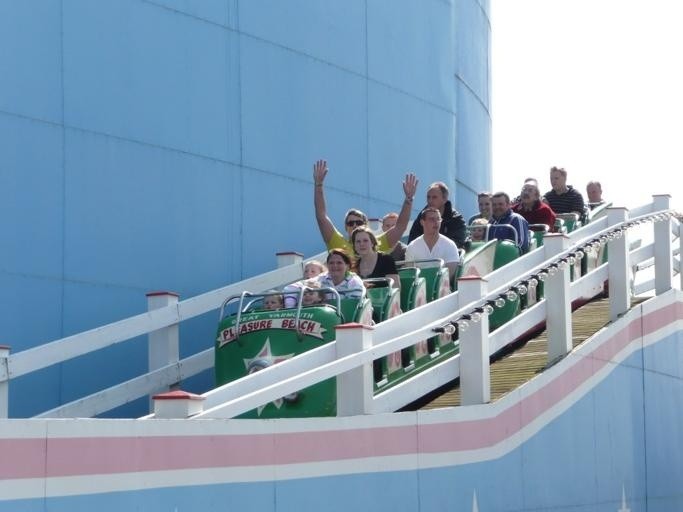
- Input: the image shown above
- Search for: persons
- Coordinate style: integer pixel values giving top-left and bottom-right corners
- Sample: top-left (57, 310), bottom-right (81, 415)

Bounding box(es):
top-left (586, 181), bottom-right (603, 209)
top-left (303, 290), bottom-right (325, 306)
top-left (381, 213), bottom-right (407, 261)
top-left (512, 182), bottom-right (556, 232)
top-left (283, 248), bottom-right (366, 309)
top-left (313, 159), bottom-right (419, 269)
top-left (511, 178), bottom-right (550, 206)
top-left (542, 165), bottom-right (583, 219)
top-left (466, 218), bottom-right (490, 245)
top-left (484, 193), bottom-right (530, 253)
top-left (350, 226), bottom-right (401, 294)
top-left (468, 193), bottom-right (494, 225)
top-left (408, 182), bottom-right (471, 249)
top-left (405, 207), bottom-right (459, 281)
top-left (304, 261), bottom-right (323, 280)
top-left (264, 290), bottom-right (282, 310)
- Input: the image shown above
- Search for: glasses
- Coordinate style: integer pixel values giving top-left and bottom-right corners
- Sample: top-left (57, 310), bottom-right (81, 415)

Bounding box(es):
top-left (346, 220), bottom-right (363, 226)
top-left (437, 218), bottom-right (443, 222)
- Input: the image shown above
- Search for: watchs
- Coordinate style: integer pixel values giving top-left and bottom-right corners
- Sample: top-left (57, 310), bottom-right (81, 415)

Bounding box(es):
top-left (314, 183), bottom-right (322, 188)
